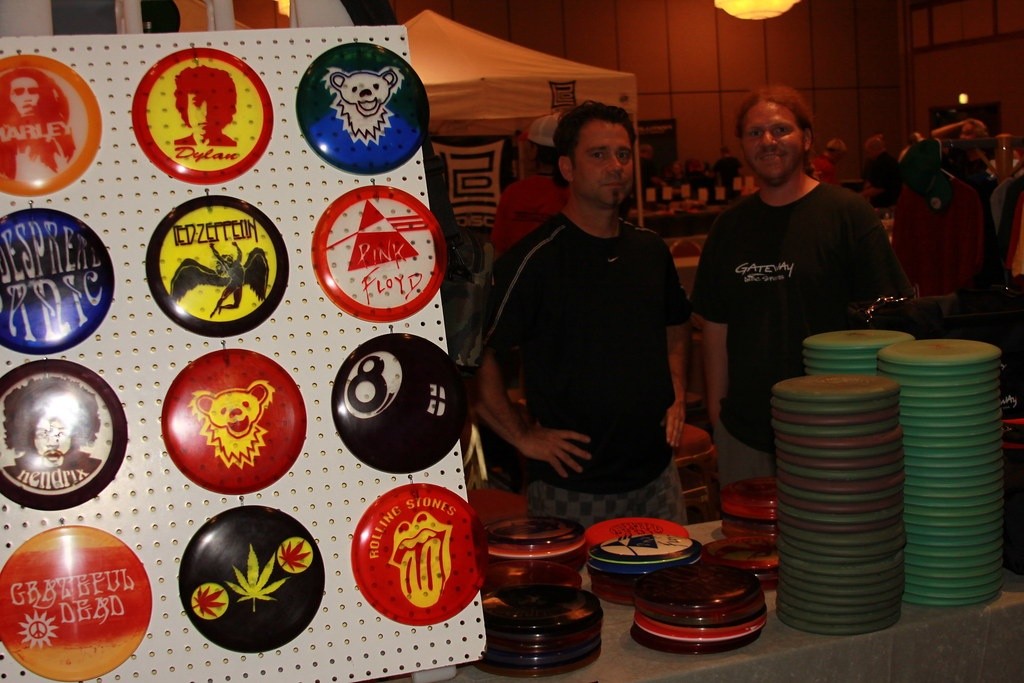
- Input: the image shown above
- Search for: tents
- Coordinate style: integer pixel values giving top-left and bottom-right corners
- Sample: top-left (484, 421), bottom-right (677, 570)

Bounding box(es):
top-left (405, 9), bottom-right (644, 231)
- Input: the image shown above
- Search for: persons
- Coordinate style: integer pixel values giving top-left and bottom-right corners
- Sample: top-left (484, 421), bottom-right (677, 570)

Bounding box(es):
top-left (814, 119), bottom-right (1024, 304)
top-left (698, 87), bottom-right (913, 480)
top-left (492, 111), bottom-right (571, 255)
top-left (638, 140), bottom-right (744, 206)
top-left (470, 98), bottom-right (694, 530)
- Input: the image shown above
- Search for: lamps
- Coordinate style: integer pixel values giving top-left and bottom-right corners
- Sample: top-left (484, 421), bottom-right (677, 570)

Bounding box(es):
top-left (714, 0), bottom-right (801, 20)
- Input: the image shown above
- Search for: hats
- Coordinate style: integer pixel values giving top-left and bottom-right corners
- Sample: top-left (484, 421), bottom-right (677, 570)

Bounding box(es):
top-left (528, 112), bottom-right (560, 146)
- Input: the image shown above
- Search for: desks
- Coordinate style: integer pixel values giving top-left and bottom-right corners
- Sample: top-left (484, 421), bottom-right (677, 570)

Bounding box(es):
top-left (660, 234), bottom-right (709, 279)
top-left (625, 202), bottom-right (727, 235)
top-left (444, 517), bottom-right (1024, 682)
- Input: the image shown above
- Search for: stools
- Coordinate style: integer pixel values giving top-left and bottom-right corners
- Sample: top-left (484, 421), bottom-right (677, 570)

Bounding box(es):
top-left (673, 423), bottom-right (723, 524)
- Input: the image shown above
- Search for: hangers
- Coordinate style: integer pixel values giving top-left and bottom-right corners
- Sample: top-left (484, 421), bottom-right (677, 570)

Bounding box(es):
top-left (933, 137), bottom-right (1024, 179)
